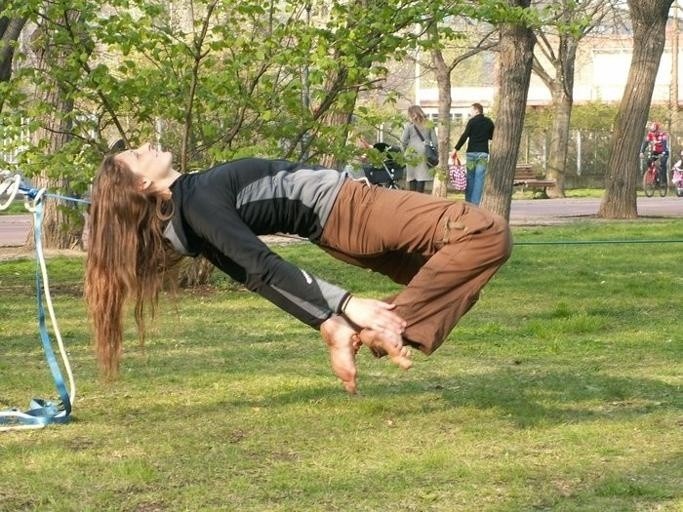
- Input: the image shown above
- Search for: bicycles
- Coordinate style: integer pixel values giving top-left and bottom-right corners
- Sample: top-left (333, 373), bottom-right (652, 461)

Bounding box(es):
top-left (639, 150), bottom-right (668, 197)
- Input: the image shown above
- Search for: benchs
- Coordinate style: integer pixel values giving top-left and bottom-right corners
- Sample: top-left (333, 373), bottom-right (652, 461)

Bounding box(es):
top-left (513, 165), bottom-right (557, 198)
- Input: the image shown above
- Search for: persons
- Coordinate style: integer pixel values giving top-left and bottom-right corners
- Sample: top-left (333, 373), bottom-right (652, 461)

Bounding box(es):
top-left (640, 122), bottom-right (670, 190)
top-left (78, 143), bottom-right (513, 398)
top-left (399, 105), bottom-right (439, 192)
top-left (669, 151), bottom-right (683, 191)
top-left (450, 103), bottom-right (494, 207)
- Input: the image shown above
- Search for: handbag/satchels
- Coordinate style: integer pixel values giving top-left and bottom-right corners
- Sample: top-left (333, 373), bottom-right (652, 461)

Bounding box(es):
top-left (446, 156), bottom-right (469, 191)
top-left (424, 143), bottom-right (441, 164)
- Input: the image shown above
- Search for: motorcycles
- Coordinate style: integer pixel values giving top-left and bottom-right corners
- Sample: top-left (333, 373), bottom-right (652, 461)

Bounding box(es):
top-left (671, 167), bottom-right (683, 197)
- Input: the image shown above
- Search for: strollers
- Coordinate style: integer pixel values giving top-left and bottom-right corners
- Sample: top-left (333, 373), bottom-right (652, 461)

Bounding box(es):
top-left (358, 136), bottom-right (409, 192)
